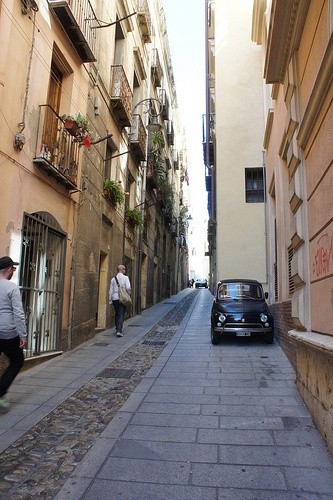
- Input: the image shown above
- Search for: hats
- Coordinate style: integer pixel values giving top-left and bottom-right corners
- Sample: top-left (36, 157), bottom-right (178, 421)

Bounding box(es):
top-left (0, 256), bottom-right (20, 269)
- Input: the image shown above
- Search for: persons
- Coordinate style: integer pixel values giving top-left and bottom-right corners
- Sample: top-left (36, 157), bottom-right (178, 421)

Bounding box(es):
top-left (0, 256), bottom-right (27, 410)
top-left (108, 265), bottom-right (131, 337)
top-left (189, 279), bottom-right (194, 288)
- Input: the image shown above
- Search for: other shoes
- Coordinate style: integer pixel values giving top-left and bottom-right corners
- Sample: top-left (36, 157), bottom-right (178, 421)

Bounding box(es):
top-left (116, 332), bottom-right (124, 337)
top-left (0, 396), bottom-right (12, 408)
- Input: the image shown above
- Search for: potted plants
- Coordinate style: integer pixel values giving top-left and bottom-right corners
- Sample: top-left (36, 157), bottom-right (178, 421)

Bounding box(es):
top-left (125, 209), bottom-right (142, 227)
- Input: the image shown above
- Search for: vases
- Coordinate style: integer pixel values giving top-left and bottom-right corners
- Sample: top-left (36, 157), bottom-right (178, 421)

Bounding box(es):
top-left (64, 120), bottom-right (78, 136)
top-left (104, 188), bottom-right (114, 202)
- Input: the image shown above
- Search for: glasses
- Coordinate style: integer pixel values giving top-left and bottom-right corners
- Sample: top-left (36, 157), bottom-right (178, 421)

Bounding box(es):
top-left (123, 268), bottom-right (127, 270)
top-left (11, 267), bottom-right (16, 271)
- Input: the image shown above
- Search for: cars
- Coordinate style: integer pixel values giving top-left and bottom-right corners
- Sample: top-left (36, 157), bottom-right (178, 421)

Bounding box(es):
top-left (210, 278), bottom-right (275, 347)
top-left (195, 278), bottom-right (207, 289)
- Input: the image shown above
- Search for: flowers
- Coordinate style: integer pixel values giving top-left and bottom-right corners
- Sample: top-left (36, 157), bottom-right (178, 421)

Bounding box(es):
top-left (56, 114), bottom-right (94, 148)
top-left (103, 177), bottom-right (125, 205)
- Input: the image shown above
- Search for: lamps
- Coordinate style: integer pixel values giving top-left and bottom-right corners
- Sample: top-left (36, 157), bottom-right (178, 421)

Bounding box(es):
top-left (133, 98), bottom-right (163, 131)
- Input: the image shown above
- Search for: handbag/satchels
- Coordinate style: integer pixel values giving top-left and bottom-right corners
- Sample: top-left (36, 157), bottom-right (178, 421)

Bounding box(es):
top-left (118, 287), bottom-right (133, 307)
